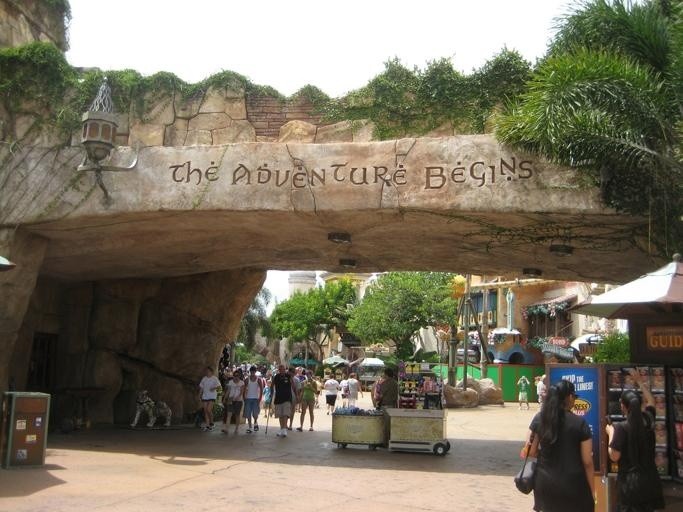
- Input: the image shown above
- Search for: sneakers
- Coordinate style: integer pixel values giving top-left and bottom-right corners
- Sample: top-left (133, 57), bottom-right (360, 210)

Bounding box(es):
top-left (221, 429), bottom-right (228, 434)
top-left (297, 427), bottom-right (303, 432)
top-left (282, 429), bottom-right (288, 438)
top-left (288, 428), bottom-right (292, 431)
top-left (309, 429), bottom-right (313, 432)
top-left (277, 431), bottom-right (281, 437)
top-left (202, 426), bottom-right (212, 433)
top-left (234, 427), bottom-right (240, 434)
top-left (209, 423), bottom-right (215, 427)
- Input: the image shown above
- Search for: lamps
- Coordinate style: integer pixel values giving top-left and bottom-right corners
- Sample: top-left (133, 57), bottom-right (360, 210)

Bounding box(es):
top-left (76, 72), bottom-right (138, 206)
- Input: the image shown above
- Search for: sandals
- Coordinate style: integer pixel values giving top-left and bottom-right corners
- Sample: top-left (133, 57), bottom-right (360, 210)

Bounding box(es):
top-left (246, 428), bottom-right (253, 433)
top-left (253, 425), bottom-right (259, 431)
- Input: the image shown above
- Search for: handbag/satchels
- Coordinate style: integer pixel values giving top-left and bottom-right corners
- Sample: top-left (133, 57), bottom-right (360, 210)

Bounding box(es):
top-left (514, 460), bottom-right (538, 495)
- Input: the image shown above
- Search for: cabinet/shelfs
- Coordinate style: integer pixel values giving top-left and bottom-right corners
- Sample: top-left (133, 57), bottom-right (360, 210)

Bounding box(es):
top-left (670, 366), bottom-right (683, 482)
top-left (605, 368), bottom-right (669, 481)
top-left (397, 362), bottom-right (438, 409)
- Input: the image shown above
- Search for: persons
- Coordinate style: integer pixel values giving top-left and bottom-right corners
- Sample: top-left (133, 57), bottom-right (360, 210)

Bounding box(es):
top-left (368, 368), bottom-right (401, 412)
top-left (196, 359), bottom-right (363, 436)
top-left (517, 376), bottom-right (530, 410)
top-left (534, 374), bottom-right (547, 403)
top-left (524, 380), bottom-right (596, 512)
top-left (605, 366), bottom-right (666, 512)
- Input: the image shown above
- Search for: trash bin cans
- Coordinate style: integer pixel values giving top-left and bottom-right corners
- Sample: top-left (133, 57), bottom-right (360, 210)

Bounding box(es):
top-left (1, 392), bottom-right (52, 469)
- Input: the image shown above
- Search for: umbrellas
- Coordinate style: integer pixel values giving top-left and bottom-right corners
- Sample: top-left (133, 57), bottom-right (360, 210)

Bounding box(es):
top-left (559, 253), bottom-right (683, 320)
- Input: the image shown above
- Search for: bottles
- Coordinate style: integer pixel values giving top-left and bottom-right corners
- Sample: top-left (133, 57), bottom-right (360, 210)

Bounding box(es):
top-left (399, 378), bottom-right (404, 393)
top-left (405, 377), bottom-right (409, 392)
top-left (412, 378), bottom-right (415, 391)
